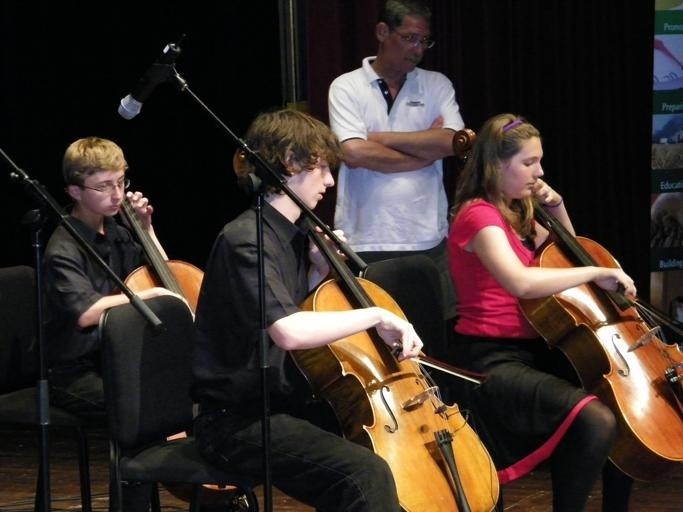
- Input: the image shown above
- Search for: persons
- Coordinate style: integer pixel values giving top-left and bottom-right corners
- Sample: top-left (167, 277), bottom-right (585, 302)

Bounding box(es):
top-left (447, 113), bottom-right (637, 512)
top-left (189, 107), bottom-right (425, 512)
top-left (328, 0), bottom-right (466, 363)
top-left (39, 136), bottom-right (195, 512)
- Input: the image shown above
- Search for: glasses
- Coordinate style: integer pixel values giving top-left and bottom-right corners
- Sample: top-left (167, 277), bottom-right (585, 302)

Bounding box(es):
top-left (393, 29), bottom-right (436, 49)
top-left (83, 178), bottom-right (130, 194)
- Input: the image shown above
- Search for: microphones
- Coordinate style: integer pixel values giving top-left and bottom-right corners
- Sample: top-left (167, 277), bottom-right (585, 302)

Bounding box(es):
top-left (117, 33), bottom-right (187, 120)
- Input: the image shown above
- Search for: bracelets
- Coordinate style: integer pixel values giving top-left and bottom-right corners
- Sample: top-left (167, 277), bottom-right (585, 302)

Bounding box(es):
top-left (543, 194), bottom-right (563, 208)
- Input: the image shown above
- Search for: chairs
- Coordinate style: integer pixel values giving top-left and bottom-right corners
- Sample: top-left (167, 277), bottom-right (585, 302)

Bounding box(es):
top-left (1, 264), bottom-right (92, 511)
top-left (100, 295), bottom-right (264, 512)
top-left (358, 254), bottom-right (453, 406)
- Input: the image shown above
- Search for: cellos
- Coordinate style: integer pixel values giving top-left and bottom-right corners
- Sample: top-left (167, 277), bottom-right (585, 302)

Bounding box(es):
top-left (233, 139), bottom-right (499, 512)
top-left (451, 128), bottom-right (683, 486)
top-left (112, 193), bottom-right (237, 504)
